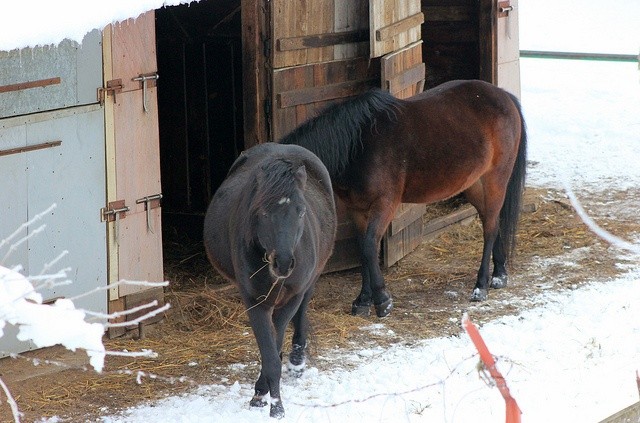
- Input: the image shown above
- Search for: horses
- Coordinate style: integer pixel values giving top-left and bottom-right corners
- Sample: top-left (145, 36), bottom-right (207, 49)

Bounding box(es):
top-left (277, 79), bottom-right (528, 318)
top-left (203, 141), bottom-right (338, 420)
